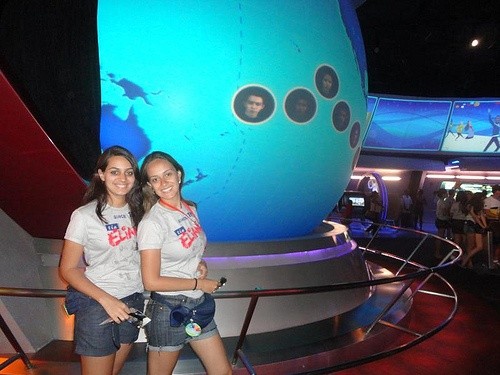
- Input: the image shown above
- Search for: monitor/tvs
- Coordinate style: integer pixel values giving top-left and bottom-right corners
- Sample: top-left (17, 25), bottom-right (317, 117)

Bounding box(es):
top-left (349, 197), bottom-right (364, 207)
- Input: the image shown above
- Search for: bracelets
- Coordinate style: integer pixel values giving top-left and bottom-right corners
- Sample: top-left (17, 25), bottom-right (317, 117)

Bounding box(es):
top-left (193, 278), bottom-right (198, 291)
top-left (136, 151), bottom-right (233, 375)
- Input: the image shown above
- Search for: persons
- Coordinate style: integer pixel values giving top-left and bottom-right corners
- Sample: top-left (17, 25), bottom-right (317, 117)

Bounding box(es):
top-left (239, 91), bottom-right (271, 123)
top-left (445, 118), bottom-right (475, 140)
top-left (317, 69), bottom-right (337, 98)
top-left (399, 190), bottom-right (425, 231)
top-left (435, 185), bottom-right (500, 269)
top-left (364, 192), bottom-right (383, 239)
top-left (289, 94), bottom-right (314, 123)
top-left (340, 194), bottom-right (354, 232)
top-left (334, 105), bottom-right (349, 131)
top-left (60, 145), bottom-right (208, 375)
top-left (482, 108), bottom-right (500, 152)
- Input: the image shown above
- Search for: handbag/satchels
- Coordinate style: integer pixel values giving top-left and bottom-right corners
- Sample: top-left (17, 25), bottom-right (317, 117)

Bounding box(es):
top-left (154, 293), bottom-right (215, 331)
top-left (62, 286), bottom-right (87, 318)
